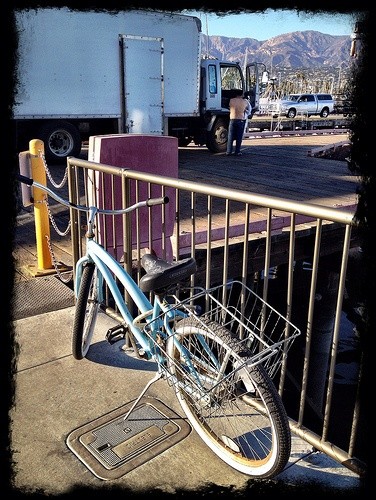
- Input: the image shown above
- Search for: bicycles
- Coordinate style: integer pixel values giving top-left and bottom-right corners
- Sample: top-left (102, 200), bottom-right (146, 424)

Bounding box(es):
top-left (18, 175), bottom-right (301, 479)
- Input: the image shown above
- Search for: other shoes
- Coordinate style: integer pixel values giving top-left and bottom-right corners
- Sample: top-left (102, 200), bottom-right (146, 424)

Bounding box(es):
top-left (225, 152), bottom-right (232, 156)
top-left (235, 152), bottom-right (243, 155)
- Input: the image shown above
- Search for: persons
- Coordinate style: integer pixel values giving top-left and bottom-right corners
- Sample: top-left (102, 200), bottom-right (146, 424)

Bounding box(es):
top-left (225, 89), bottom-right (248, 156)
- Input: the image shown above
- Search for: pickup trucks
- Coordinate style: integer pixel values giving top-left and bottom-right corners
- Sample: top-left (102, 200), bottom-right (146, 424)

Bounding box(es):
top-left (269, 93), bottom-right (334, 118)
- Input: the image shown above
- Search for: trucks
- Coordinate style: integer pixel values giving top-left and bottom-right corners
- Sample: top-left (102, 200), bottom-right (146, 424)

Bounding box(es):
top-left (13, 7), bottom-right (269, 165)
